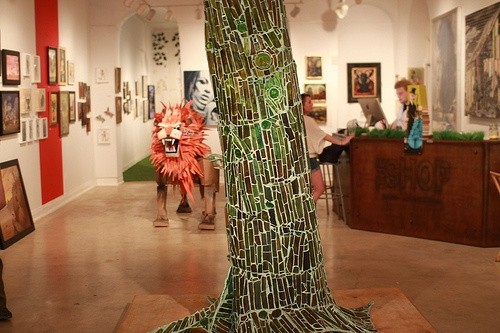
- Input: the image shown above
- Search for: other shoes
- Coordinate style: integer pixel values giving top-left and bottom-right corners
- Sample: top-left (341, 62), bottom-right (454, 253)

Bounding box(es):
top-left (0, 308), bottom-right (12, 320)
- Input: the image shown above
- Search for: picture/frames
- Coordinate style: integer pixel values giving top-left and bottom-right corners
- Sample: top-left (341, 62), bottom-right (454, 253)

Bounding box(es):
top-left (0, 158), bottom-right (35, 250)
top-left (305, 84), bottom-right (327, 102)
top-left (305, 56), bottom-right (323, 79)
top-left (184, 70), bottom-right (219, 126)
top-left (305, 106), bottom-right (326, 124)
top-left (347, 63), bottom-right (381, 104)
top-left (0, 46), bottom-right (155, 143)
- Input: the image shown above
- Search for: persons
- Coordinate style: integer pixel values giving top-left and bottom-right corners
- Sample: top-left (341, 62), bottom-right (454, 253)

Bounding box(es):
top-left (301, 94), bottom-right (354, 205)
top-left (0, 258), bottom-right (13, 319)
top-left (185, 71), bottom-right (219, 125)
top-left (394, 79), bottom-right (413, 131)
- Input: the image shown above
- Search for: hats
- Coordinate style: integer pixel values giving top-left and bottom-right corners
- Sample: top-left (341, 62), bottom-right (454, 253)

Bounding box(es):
top-left (409, 87), bottom-right (416, 95)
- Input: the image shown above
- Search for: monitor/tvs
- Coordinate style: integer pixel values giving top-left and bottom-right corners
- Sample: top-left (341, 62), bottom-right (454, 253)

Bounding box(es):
top-left (358, 98), bottom-right (389, 131)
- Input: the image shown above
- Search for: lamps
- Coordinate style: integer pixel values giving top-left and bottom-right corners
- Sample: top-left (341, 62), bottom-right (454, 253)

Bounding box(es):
top-left (135, 0), bottom-right (350, 21)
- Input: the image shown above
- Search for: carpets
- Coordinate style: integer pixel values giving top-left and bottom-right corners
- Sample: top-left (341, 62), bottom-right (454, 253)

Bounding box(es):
top-left (123, 153), bottom-right (202, 182)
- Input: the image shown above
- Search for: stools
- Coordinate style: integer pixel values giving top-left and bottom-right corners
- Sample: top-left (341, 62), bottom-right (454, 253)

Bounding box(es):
top-left (319, 160), bottom-right (346, 224)
